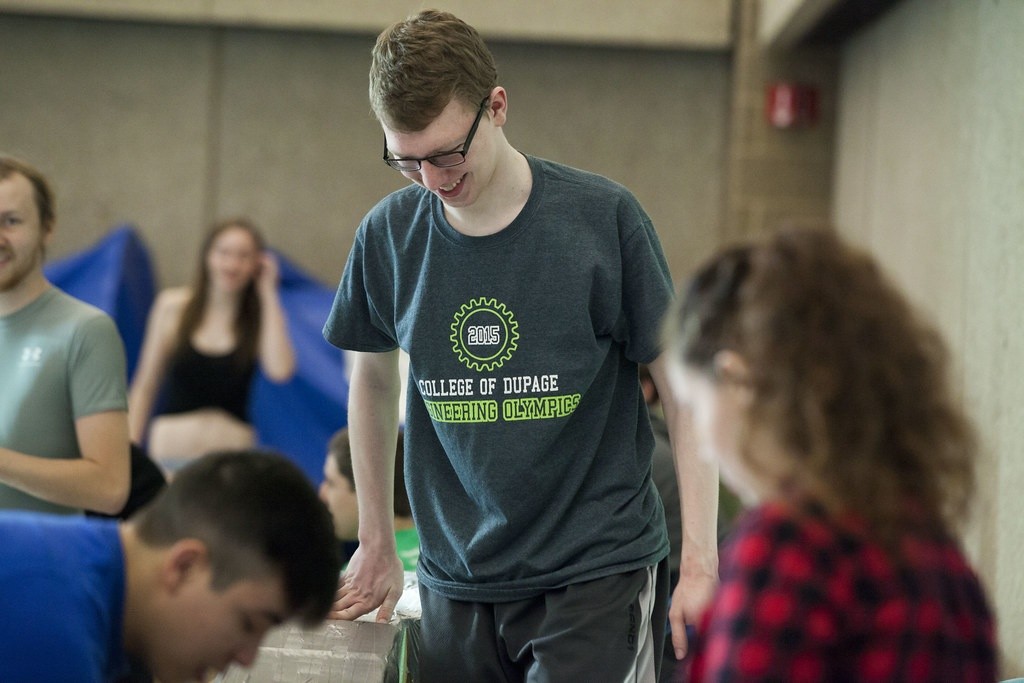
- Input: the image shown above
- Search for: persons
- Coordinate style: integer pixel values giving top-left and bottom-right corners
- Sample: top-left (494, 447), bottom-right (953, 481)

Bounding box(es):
top-left (319, 8), bottom-right (720, 683)
top-left (0, 153), bottom-right (419, 683)
top-left (665, 222), bottom-right (1003, 683)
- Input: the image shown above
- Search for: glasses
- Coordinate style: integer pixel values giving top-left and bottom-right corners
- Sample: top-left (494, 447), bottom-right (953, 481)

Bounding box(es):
top-left (382, 96), bottom-right (489, 172)
